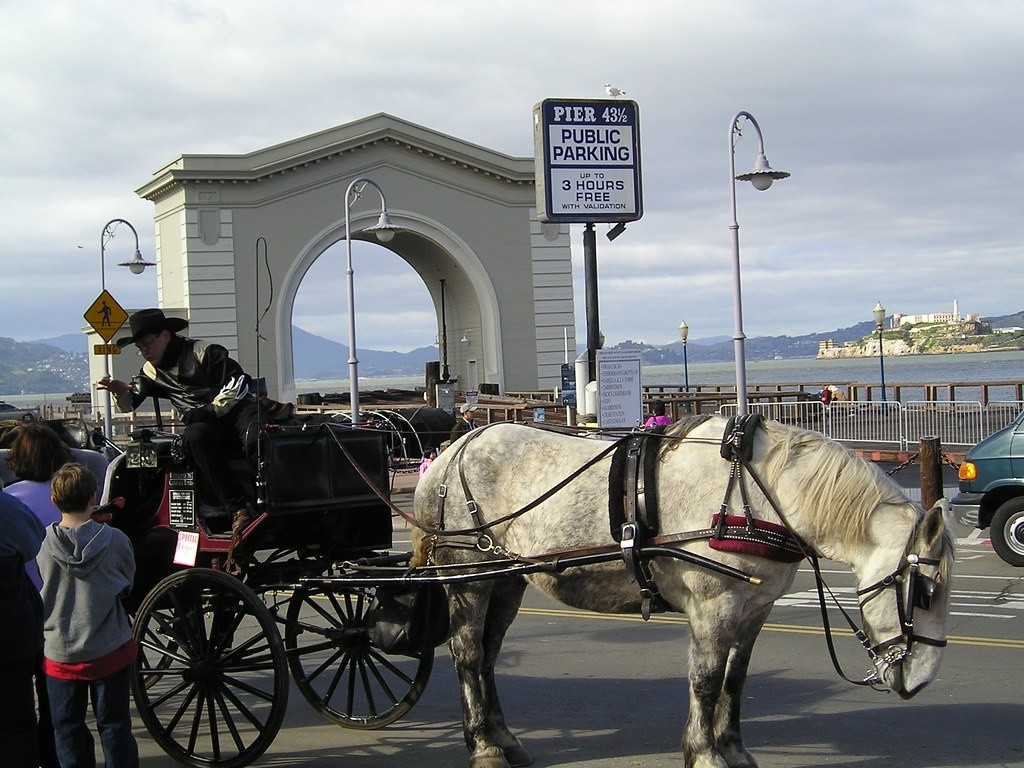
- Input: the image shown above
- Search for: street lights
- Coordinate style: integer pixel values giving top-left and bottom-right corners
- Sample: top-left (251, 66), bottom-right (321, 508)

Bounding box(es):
top-left (678, 320), bottom-right (692, 412)
top-left (101, 218), bottom-right (156, 447)
top-left (872, 301), bottom-right (888, 415)
top-left (344, 178), bottom-right (409, 429)
top-left (729, 111), bottom-right (791, 418)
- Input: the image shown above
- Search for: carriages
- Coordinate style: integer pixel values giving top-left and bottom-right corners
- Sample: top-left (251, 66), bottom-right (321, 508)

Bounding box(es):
top-left (0, 376), bottom-right (954, 768)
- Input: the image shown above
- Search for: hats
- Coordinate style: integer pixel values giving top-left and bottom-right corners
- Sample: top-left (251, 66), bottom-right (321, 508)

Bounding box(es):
top-left (116, 308), bottom-right (189, 349)
top-left (460, 402), bottom-right (477, 413)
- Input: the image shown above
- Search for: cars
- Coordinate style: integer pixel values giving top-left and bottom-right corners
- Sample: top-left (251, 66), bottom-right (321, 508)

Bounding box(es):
top-left (0, 401), bottom-right (40, 421)
top-left (952, 410), bottom-right (1024, 567)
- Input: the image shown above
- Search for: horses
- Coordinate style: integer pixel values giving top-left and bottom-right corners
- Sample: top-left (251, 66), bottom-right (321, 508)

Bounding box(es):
top-left (408, 414), bottom-right (962, 768)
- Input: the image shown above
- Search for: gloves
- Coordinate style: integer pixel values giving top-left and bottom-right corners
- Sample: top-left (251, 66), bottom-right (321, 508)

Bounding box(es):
top-left (182, 406), bottom-right (217, 425)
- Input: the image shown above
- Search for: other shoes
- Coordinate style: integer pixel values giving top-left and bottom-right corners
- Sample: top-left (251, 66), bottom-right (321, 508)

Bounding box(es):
top-left (232, 509), bottom-right (250, 530)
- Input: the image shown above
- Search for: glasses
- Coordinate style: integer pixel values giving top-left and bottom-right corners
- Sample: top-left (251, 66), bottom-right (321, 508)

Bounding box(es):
top-left (136, 333), bottom-right (159, 356)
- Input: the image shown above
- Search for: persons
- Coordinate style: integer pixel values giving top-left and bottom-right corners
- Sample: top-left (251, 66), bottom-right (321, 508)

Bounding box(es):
top-left (818, 384), bottom-right (847, 405)
top-left (419, 446), bottom-right (437, 479)
top-left (95, 307), bottom-right (296, 533)
top-left (0, 421), bottom-right (139, 768)
top-left (646, 400), bottom-right (671, 427)
top-left (450, 403), bottom-right (479, 447)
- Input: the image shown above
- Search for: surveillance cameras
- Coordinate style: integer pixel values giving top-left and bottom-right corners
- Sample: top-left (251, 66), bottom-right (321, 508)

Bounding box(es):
top-left (607, 224), bottom-right (627, 241)
